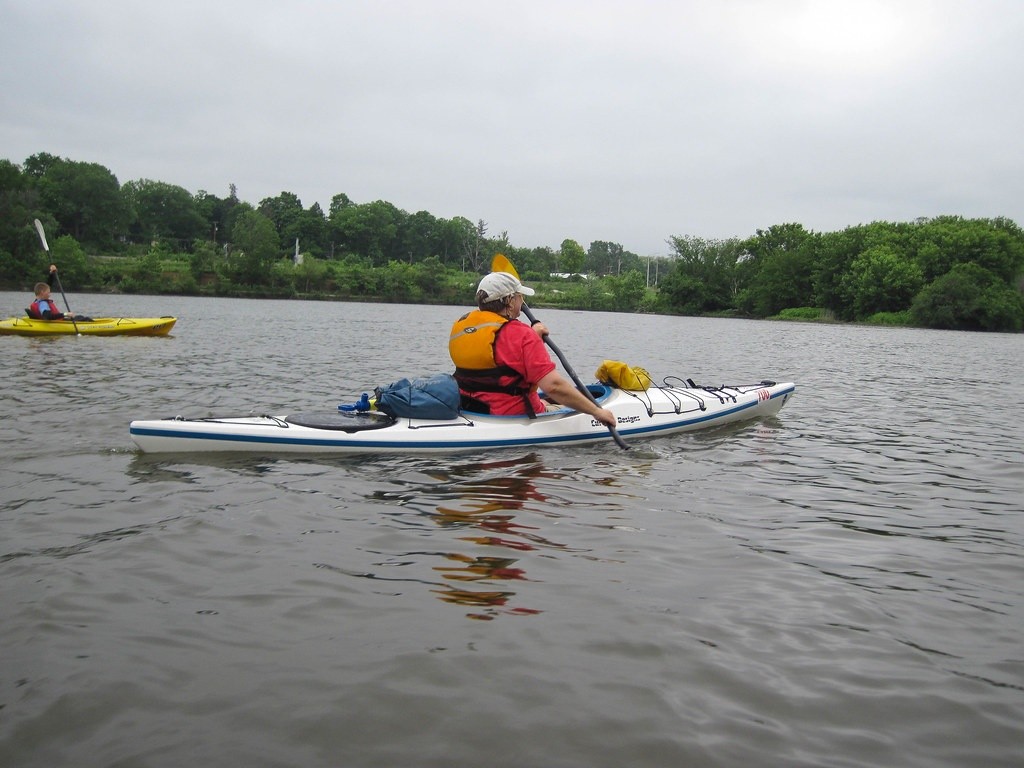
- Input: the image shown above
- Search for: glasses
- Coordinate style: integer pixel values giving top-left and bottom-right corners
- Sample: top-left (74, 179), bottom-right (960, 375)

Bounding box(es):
top-left (511, 294), bottom-right (525, 300)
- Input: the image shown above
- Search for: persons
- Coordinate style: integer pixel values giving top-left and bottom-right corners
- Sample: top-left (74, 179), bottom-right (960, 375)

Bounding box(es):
top-left (449, 272), bottom-right (616, 427)
top-left (30, 265), bottom-right (74, 320)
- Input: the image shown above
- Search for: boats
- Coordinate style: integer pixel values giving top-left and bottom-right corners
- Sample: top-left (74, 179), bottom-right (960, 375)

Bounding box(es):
top-left (0, 314), bottom-right (179, 337)
top-left (129, 358), bottom-right (799, 455)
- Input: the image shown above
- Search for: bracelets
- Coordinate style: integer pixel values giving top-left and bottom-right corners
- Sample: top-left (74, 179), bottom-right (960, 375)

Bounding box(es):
top-left (63, 312), bottom-right (68, 317)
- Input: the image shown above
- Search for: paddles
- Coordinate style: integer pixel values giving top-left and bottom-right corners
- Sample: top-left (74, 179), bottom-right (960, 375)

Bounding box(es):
top-left (34, 218), bottom-right (81, 337)
top-left (492, 253), bottom-right (634, 452)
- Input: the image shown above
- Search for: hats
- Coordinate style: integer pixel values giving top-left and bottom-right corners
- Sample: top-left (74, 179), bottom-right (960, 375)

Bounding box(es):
top-left (476, 272), bottom-right (535, 303)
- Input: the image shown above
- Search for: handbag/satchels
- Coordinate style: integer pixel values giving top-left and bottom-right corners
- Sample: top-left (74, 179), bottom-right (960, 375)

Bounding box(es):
top-left (374, 373), bottom-right (460, 419)
